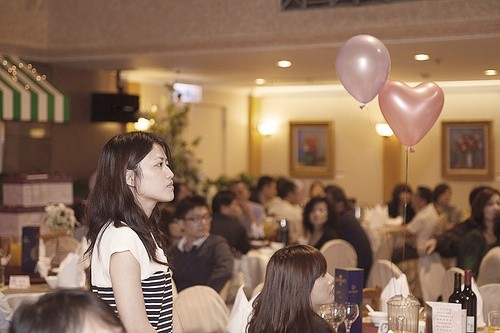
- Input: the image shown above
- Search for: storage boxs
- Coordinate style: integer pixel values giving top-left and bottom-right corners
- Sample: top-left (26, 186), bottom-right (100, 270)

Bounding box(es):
top-left (334, 268), bottom-right (365, 333)
top-left (21, 225), bottom-right (40, 277)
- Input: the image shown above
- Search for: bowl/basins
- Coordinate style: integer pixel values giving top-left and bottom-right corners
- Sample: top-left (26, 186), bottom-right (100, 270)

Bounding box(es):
top-left (367, 311), bottom-right (388, 326)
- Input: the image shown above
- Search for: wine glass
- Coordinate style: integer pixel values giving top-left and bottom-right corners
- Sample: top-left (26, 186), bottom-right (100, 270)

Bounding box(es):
top-left (316, 301), bottom-right (359, 333)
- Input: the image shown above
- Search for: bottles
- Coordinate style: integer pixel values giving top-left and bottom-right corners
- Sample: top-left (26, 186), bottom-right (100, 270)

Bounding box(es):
top-left (462, 270), bottom-right (477, 333)
top-left (448, 272), bottom-right (468, 333)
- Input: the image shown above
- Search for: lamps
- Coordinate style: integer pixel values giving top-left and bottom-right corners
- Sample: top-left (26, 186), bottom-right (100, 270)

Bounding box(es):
top-left (375, 122), bottom-right (394, 137)
top-left (134, 117), bottom-right (155, 131)
top-left (256, 123), bottom-right (277, 137)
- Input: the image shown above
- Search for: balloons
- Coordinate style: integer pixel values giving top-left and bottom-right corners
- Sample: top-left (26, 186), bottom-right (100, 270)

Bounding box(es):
top-left (337, 34), bottom-right (390, 104)
top-left (380, 79), bottom-right (444, 146)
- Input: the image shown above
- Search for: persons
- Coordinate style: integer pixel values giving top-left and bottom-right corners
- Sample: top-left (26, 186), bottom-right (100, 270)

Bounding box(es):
top-left (9, 289), bottom-right (126, 333)
top-left (84, 131), bottom-right (173, 333)
top-left (158, 183), bottom-right (234, 294)
top-left (210, 189), bottom-right (251, 258)
top-left (229, 180), bottom-right (263, 239)
top-left (267, 176), bottom-right (373, 289)
top-left (388, 183), bottom-right (500, 281)
top-left (250, 175), bottom-right (276, 205)
top-left (228, 245), bottom-right (336, 333)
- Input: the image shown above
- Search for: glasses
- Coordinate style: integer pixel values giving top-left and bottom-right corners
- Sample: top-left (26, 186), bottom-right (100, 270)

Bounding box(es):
top-left (187, 215), bottom-right (214, 224)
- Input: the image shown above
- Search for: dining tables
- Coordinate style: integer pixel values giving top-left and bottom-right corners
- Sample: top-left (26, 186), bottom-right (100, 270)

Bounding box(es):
top-left (1, 282), bottom-right (56, 330)
top-left (361, 310), bottom-right (489, 333)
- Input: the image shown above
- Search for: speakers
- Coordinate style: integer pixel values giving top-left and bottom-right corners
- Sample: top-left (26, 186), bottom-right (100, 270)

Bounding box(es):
top-left (91, 93), bottom-right (139, 122)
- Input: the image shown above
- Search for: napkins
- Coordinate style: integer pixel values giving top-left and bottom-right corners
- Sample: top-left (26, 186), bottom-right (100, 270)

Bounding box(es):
top-left (461, 276), bottom-right (487, 329)
top-left (379, 273), bottom-right (410, 312)
top-left (225, 282), bottom-right (261, 333)
top-left (32, 238), bottom-right (55, 279)
top-left (56, 252), bottom-right (87, 289)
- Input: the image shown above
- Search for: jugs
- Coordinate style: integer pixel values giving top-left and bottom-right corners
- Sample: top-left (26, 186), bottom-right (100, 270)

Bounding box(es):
top-left (386, 294), bottom-right (419, 333)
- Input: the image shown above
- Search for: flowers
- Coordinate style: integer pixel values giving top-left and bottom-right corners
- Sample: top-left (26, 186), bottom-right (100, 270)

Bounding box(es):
top-left (43, 201), bottom-right (81, 239)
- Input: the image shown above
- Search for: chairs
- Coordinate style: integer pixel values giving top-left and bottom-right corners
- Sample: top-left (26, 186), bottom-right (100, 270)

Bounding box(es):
top-left (43, 218), bottom-right (500, 333)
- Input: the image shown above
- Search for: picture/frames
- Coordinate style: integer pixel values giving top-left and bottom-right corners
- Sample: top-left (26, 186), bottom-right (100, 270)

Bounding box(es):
top-left (440, 119), bottom-right (496, 183)
top-left (288, 121), bottom-right (336, 180)
